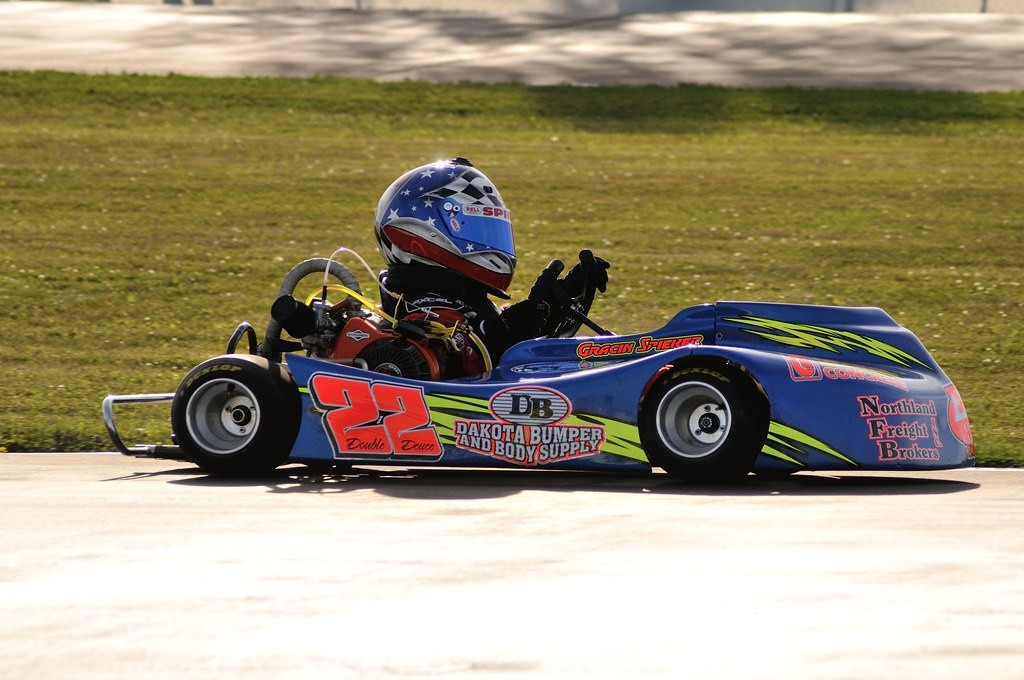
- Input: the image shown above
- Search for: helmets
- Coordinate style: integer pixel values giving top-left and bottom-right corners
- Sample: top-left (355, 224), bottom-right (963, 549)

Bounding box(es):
top-left (373, 156), bottom-right (516, 300)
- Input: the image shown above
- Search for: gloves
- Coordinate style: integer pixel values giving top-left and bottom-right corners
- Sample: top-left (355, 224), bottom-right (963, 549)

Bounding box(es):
top-left (557, 250), bottom-right (610, 336)
top-left (501, 258), bottom-right (577, 340)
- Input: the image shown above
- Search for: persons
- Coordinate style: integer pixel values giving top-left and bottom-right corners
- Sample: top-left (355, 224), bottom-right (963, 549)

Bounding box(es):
top-left (373, 154), bottom-right (610, 369)
